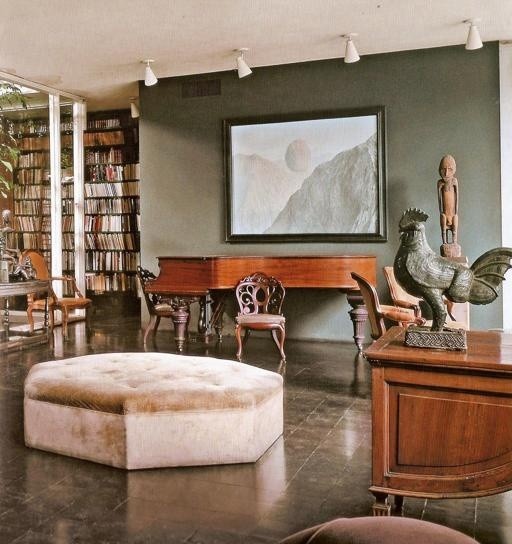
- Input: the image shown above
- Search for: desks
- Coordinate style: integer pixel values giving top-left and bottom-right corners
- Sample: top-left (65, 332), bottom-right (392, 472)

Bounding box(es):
top-left (0, 280), bottom-right (49, 354)
top-left (363, 326), bottom-right (512, 516)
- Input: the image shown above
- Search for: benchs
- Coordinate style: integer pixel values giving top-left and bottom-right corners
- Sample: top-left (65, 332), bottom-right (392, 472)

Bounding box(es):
top-left (23, 352), bottom-right (283, 470)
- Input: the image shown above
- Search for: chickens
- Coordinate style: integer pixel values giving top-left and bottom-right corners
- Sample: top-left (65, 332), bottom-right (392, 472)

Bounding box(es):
top-left (392, 206), bottom-right (512, 333)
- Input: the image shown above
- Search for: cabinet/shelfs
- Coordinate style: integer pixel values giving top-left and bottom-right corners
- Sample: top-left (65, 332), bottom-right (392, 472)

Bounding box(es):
top-left (12, 108), bottom-right (140, 314)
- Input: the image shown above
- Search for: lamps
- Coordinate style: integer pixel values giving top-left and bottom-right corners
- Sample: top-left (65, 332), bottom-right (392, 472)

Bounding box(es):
top-left (128, 97), bottom-right (140, 118)
top-left (344, 33), bottom-right (360, 64)
top-left (464, 17), bottom-right (483, 51)
top-left (141, 60), bottom-right (158, 86)
top-left (233, 48), bottom-right (253, 79)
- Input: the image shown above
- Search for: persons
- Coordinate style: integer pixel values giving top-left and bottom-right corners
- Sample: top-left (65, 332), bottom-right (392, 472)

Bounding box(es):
top-left (436, 155), bottom-right (460, 245)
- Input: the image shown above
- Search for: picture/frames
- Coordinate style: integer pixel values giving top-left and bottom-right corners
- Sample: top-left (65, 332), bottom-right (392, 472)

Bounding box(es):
top-left (223, 106), bottom-right (388, 243)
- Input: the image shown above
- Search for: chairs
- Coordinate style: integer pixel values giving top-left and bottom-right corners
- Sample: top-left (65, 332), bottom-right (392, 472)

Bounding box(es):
top-left (351, 272), bottom-right (426, 344)
top-left (19, 249), bottom-right (92, 342)
top-left (235, 272), bottom-right (286, 363)
top-left (382, 266), bottom-right (465, 329)
top-left (136, 266), bottom-right (191, 345)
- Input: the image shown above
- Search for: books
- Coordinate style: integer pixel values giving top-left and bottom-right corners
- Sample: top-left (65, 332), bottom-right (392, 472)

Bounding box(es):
top-left (10, 117), bottom-right (139, 294)
